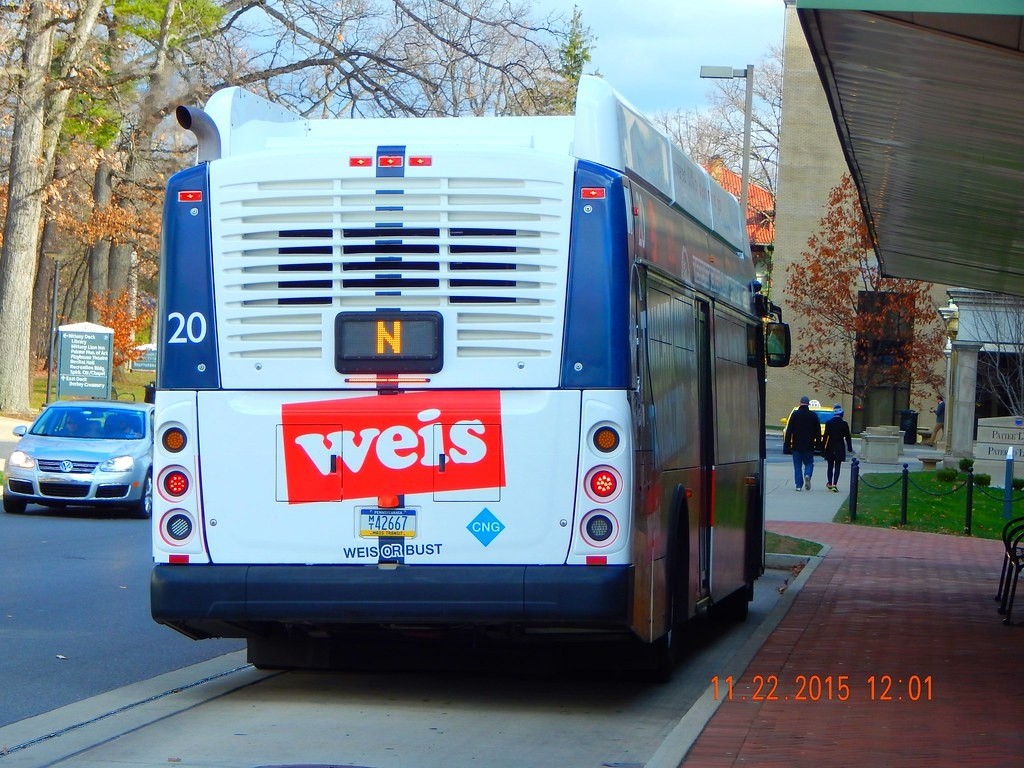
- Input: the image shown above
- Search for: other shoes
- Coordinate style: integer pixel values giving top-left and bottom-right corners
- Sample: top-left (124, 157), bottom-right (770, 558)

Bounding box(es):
top-left (796, 487), bottom-right (802, 491)
top-left (804, 475), bottom-right (811, 490)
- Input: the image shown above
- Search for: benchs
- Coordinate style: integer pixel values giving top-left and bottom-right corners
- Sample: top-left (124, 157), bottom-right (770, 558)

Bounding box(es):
top-left (994, 516), bottom-right (1024, 625)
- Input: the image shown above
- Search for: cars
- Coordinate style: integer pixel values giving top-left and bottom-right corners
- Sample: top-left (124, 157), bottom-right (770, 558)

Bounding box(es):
top-left (781, 399), bottom-right (834, 455)
top-left (2, 399), bottom-right (152, 520)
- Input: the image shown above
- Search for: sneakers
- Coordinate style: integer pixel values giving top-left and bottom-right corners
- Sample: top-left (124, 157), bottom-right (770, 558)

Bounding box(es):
top-left (832, 486), bottom-right (839, 492)
top-left (826, 482), bottom-right (832, 489)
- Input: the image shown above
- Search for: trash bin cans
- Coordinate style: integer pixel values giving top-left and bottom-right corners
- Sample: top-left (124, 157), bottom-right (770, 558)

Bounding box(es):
top-left (900, 411), bottom-right (919, 445)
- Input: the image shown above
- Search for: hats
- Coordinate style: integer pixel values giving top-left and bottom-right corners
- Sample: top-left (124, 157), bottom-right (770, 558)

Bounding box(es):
top-left (833, 403), bottom-right (844, 418)
top-left (800, 396), bottom-right (809, 405)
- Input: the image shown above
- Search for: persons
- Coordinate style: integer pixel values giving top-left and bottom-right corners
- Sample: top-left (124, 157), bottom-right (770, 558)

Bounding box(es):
top-left (821, 403), bottom-right (853, 492)
top-left (114, 413), bottom-right (142, 439)
top-left (925, 395), bottom-right (945, 446)
top-left (784, 395), bottom-right (821, 491)
top-left (58, 410), bottom-right (90, 436)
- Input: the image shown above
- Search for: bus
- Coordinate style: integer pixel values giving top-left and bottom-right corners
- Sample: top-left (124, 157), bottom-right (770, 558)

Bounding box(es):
top-left (151, 76), bottom-right (796, 687)
